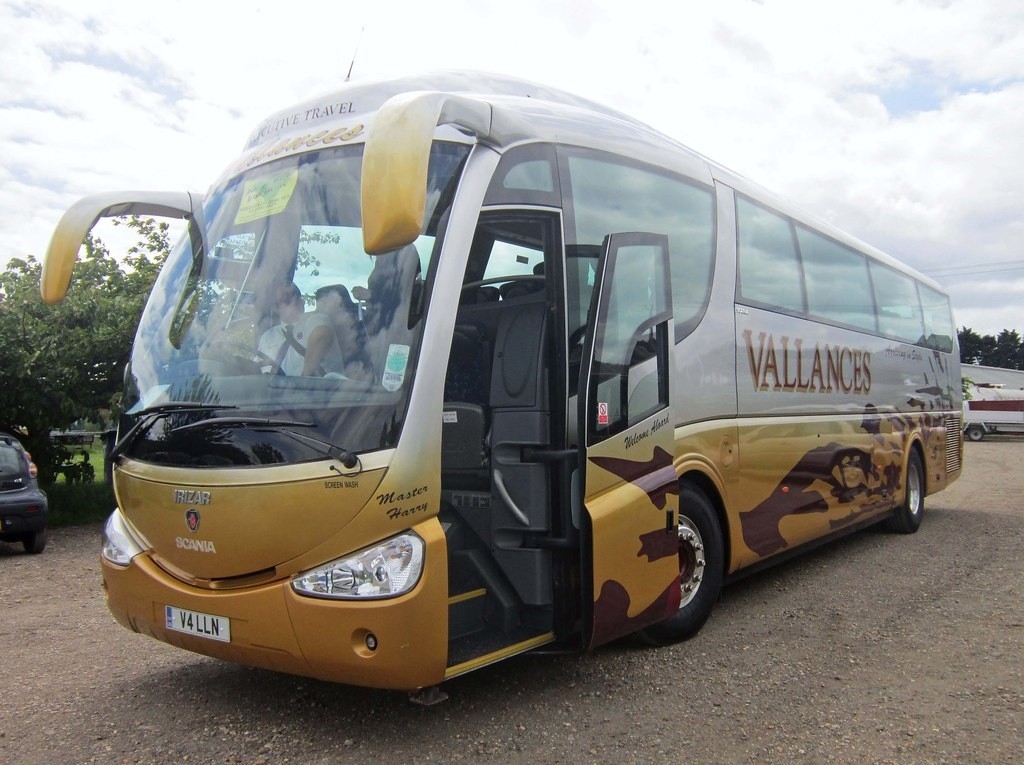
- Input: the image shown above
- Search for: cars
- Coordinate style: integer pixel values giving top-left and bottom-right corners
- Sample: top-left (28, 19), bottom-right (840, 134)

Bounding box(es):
top-left (0, 433), bottom-right (51, 555)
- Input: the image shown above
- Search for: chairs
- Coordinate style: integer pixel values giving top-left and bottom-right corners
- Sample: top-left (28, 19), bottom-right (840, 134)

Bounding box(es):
top-left (315, 262), bottom-right (589, 470)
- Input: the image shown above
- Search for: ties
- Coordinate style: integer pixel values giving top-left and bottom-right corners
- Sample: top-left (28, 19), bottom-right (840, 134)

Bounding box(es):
top-left (270, 323), bottom-right (294, 375)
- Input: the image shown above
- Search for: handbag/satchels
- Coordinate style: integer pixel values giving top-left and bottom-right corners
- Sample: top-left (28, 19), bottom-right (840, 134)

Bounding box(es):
top-left (379, 246), bottom-right (422, 330)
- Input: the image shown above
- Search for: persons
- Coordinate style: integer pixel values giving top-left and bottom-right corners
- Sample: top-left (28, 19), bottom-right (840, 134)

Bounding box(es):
top-left (251, 281), bottom-right (344, 380)
top-left (350, 241), bottom-right (422, 344)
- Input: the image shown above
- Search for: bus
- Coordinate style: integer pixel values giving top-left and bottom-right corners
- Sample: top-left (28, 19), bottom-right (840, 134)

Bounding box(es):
top-left (39, 68), bottom-right (964, 693)
top-left (959, 362), bottom-right (1024, 442)
